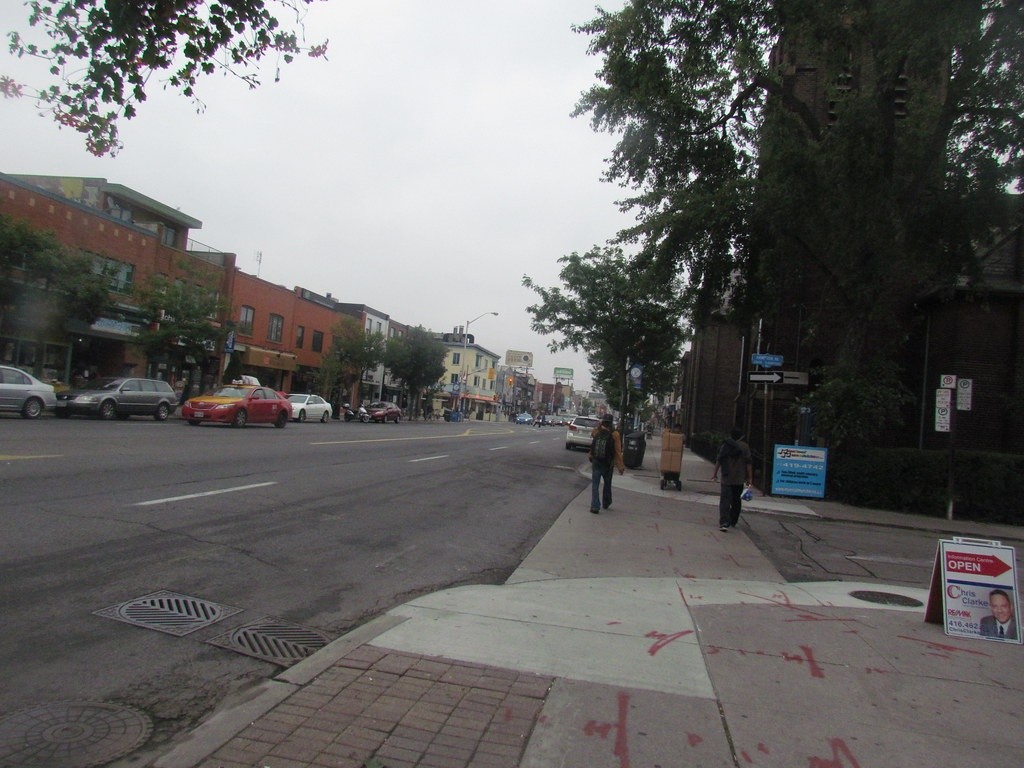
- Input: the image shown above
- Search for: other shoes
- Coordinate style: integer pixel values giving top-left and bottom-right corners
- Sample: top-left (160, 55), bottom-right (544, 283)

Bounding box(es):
top-left (603, 503), bottom-right (609, 508)
top-left (590, 509), bottom-right (599, 514)
top-left (719, 522), bottom-right (728, 531)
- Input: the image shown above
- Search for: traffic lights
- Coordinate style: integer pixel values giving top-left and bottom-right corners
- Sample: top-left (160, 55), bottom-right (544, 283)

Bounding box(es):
top-left (508, 376), bottom-right (513, 389)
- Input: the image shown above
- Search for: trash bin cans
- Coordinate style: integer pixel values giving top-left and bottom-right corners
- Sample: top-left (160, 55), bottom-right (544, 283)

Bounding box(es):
top-left (625, 431), bottom-right (647, 468)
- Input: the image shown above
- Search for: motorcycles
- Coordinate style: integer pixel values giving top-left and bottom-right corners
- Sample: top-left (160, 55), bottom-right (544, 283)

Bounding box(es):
top-left (342, 400), bottom-right (369, 423)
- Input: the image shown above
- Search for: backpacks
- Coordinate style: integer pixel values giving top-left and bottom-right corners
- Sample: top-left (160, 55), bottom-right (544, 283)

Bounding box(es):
top-left (590, 427), bottom-right (615, 464)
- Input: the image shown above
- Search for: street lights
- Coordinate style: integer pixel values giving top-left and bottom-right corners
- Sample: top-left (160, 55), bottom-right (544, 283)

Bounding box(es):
top-left (459, 312), bottom-right (499, 384)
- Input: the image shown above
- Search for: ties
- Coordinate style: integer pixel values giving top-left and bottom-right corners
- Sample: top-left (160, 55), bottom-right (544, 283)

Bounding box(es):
top-left (999, 625), bottom-right (1004, 638)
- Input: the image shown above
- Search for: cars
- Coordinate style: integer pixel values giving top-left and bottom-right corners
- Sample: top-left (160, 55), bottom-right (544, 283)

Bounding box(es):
top-left (564, 415), bottom-right (600, 451)
top-left (283, 394), bottom-right (333, 424)
top-left (536, 412), bottom-right (579, 427)
top-left (56, 376), bottom-right (180, 420)
top-left (363, 401), bottom-right (402, 423)
top-left (515, 412), bottom-right (534, 426)
top-left (0, 365), bottom-right (57, 419)
top-left (180, 379), bottom-right (293, 429)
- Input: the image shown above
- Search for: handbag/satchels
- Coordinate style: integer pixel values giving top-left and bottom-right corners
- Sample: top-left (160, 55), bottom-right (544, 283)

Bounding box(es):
top-left (740, 484), bottom-right (753, 501)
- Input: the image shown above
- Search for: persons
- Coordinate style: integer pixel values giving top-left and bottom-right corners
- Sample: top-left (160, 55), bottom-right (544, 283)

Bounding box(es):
top-left (647, 423), bottom-right (653, 432)
top-left (710, 427), bottom-right (752, 532)
top-left (980, 589), bottom-right (1018, 641)
top-left (671, 423), bottom-right (682, 434)
top-left (588, 413), bottom-right (625, 514)
